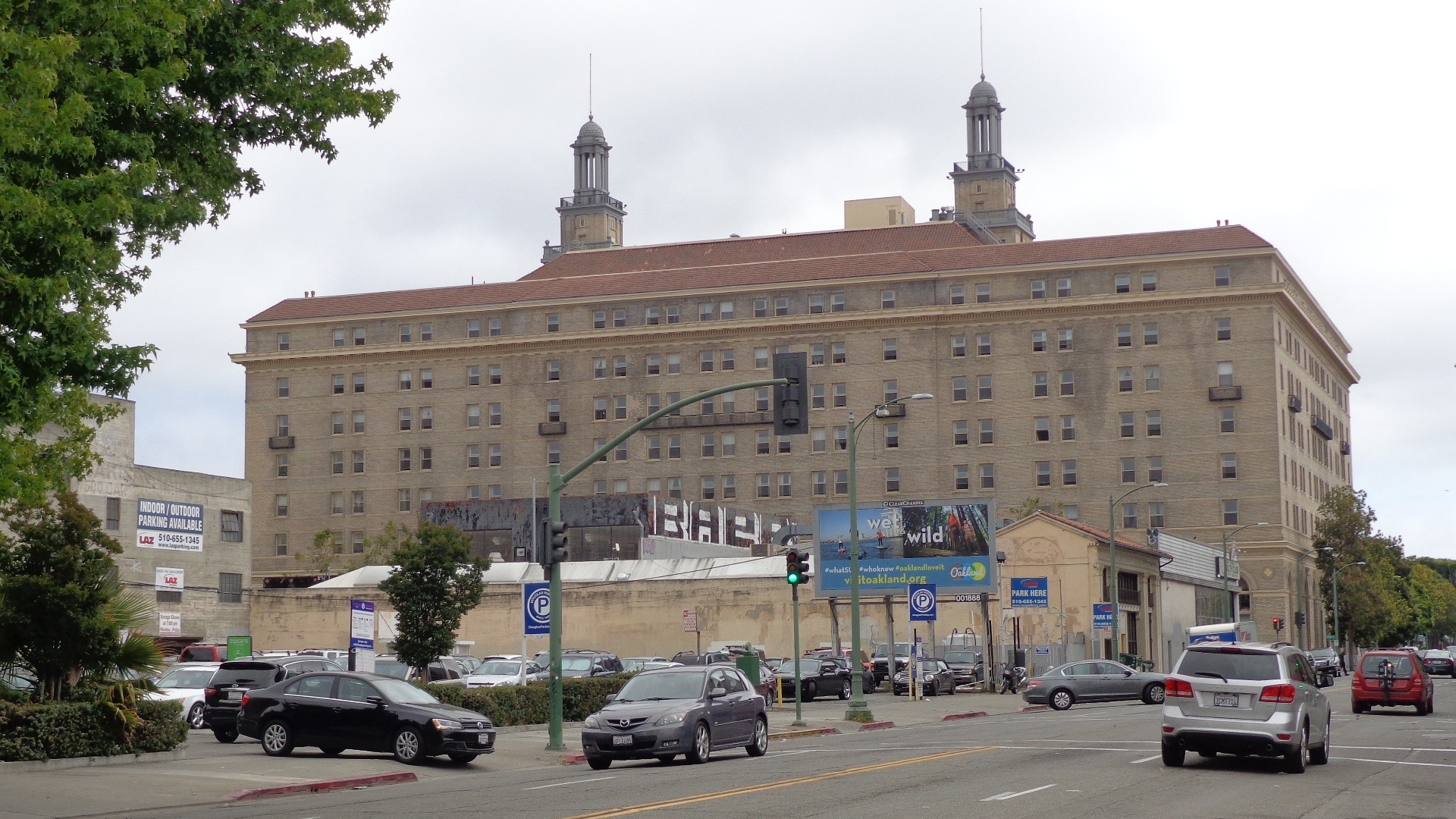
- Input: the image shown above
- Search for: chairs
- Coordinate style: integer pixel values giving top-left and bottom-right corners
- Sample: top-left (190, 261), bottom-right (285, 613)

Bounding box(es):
top-left (178, 675), bottom-right (196, 686)
top-left (292, 681), bottom-right (309, 694)
top-left (1397, 658), bottom-right (1411, 673)
top-left (1098, 665), bottom-right (1105, 674)
top-left (493, 663), bottom-right (510, 675)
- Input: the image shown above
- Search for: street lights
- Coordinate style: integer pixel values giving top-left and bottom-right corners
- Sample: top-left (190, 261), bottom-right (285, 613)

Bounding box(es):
top-left (1221, 522), bottom-right (1270, 624)
top-left (1296, 546), bottom-right (1368, 650)
top-left (846, 392), bottom-right (935, 722)
top-left (1108, 482), bottom-right (1170, 661)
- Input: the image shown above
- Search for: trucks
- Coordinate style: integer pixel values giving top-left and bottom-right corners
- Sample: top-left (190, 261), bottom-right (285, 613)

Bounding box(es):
top-left (1185, 621), bottom-right (1260, 647)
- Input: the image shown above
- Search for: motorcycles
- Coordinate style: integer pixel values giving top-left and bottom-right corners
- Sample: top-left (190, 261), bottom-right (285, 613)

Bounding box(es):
top-left (999, 661), bottom-right (1028, 695)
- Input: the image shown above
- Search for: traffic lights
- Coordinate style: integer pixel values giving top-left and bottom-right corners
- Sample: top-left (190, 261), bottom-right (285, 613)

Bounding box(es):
top-left (1272, 617), bottom-right (1284, 631)
top-left (547, 515), bottom-right (570, 566)
top-left (1297, 611), bottom-right (1307, 626)
top-left (785, 551), bottom-right (810, 585)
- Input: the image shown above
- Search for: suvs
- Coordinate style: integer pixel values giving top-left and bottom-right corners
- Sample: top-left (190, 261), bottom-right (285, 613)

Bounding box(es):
top-left (177, 641), bottom-right (625, 744)
top-left (1301, 646), bottom-right (1341, 678)
top-left (1160, 637), bottom-right (1332, 774)
top-left (671, 645), bottom-right (778, 708)
top-left (870, 642), bottom-right (929, 686)
top-left (805, 646), bottom-right (872, 673)
top-left (1351, 648), bottom-right (1434, 716)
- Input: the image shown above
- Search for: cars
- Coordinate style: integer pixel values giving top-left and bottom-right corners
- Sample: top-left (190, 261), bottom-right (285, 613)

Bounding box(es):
top-left (582, 664), bottom-right (768, 770)
top-left (138, 661), bottom-right (223, 730)
top-left (618, 656), bottom-right (687, 674)
top-left (1396, 645), bottom-right (1456, 680)
top-left (1021, 659), bottom-right (1172, 710)
top-left (766, 657), bottom-right (875, 702)
top-left (236, 671), bottom-right (498, 766)
top-left (893, 651), bottom-right (985, 696)
